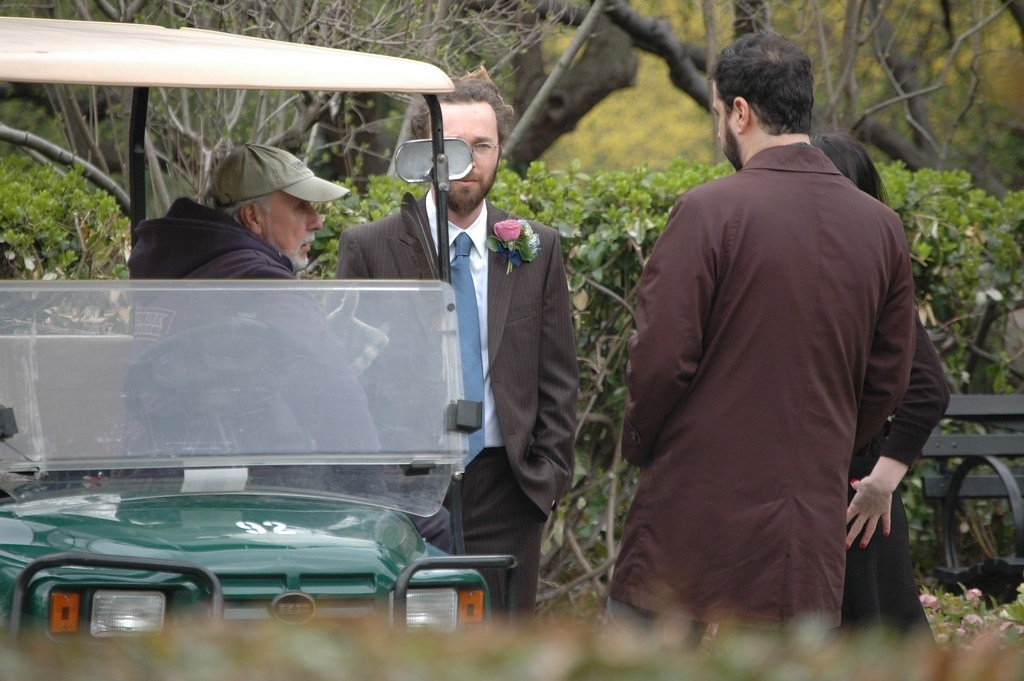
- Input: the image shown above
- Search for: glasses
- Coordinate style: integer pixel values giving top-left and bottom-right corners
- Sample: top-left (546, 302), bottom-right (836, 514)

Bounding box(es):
top-left (472, 140), bottom-right (500, 158)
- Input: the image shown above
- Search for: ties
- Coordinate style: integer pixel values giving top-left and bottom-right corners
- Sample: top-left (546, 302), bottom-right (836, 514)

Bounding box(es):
top-left (449, 231), bottom-right (486, 469)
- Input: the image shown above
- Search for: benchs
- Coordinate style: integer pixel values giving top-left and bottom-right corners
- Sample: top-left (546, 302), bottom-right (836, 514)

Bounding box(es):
top-left (920, 393), bottom-right (1024, 607)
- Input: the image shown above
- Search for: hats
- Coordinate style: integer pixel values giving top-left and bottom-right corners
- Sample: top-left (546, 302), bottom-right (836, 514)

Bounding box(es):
top-left (210, 143), bottom-right (351, 206)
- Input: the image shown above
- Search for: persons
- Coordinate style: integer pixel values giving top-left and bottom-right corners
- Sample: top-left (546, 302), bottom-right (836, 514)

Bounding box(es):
top-left (608, 38), bottom-right (915, 653)
top-left (335, 66), bottom-right (578, 622)
top-left (808, 132), bottom-right (951, 644)
top-left (128, 143), bottom-right (452, 552)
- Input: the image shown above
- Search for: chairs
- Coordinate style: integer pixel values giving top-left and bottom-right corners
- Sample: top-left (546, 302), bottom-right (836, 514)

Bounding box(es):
top-left (0, 333), bottom-right (134, 461)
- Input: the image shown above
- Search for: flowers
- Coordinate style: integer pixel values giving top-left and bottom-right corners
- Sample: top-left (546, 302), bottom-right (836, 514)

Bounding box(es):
top-left (485, 218), bottom-right (542, 275)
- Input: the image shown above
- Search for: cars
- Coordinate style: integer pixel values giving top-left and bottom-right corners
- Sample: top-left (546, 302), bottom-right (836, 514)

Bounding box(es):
top-left (0, 15), bottom-right (519, 642)
